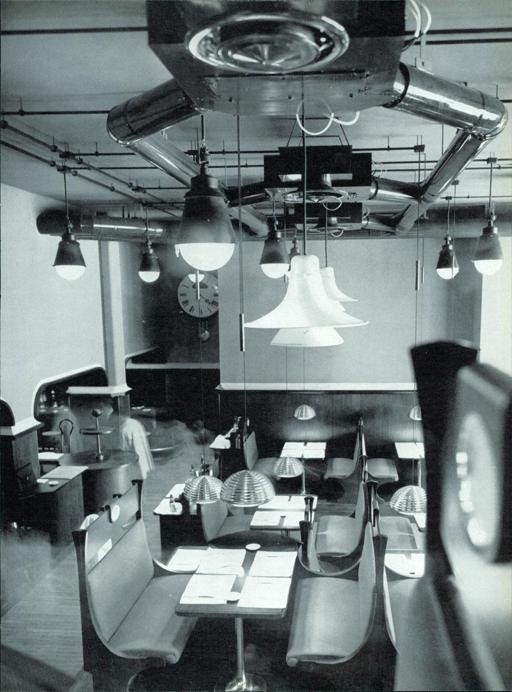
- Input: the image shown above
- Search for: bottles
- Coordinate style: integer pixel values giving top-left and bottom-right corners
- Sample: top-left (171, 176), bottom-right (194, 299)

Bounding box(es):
top-left (170, 494), bottom-right (175, 513)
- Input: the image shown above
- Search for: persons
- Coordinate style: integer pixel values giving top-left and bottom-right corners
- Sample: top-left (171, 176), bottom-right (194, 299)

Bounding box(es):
top-left (110, 409), bottom-right (153, 521)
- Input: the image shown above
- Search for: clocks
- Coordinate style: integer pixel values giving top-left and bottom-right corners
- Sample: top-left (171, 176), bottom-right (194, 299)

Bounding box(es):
top-left (175, 270), bottom-right (218, 320)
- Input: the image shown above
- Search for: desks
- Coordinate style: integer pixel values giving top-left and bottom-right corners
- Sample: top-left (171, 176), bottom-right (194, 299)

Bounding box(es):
top-left (153, 474), bottom-right (211, 555)
top-left (250, 493), bottom-right (317, 546)
top-left (174, 546), bottom-right (298, 691)
top-left (210, 419), bottom-right (238, 470)
top-left (394, 438), bottom-right (426, 487)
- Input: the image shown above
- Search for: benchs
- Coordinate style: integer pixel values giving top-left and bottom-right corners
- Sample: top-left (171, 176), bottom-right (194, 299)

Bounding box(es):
top-left (68, 479), bottom-right (199, 688)
top-left (323, 418), bottom-right (399, 505)
top-left (238, 426), bottom-right (281, 488)
top-left (314, 483), bottom-right (424, 569)
top-left (284, 520), bottom-right (419, 691)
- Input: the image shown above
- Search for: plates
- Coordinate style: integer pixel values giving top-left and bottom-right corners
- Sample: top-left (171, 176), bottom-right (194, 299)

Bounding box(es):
top-left (245, 543), bottom-right (261, 550)
top-left (224, 591), bottom-right (241, 601)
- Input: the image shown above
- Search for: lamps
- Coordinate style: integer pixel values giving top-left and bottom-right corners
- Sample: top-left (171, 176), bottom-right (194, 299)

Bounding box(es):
top-left (54, 169), bottom-right (87, 283)
top-left (245, 116), bottom-right (369, 346)
top-left (174, 115), bottom-right (238, 270)
top-left (293, 404), bottom-right (317, 422)
top-left (314, 241), bottom-right (362, 305)
top-left (388, 483), bottom-right (429, 516)
top-left (272, 454), bottom-right (304, 480)
top-left (472, 159), bottom-right (503, 277)
top-left (135, 201), bottom-right (161, 286)
top-left (409, 405), bottom-right (422, 421)
top-left (218, 466), bottom-right (275, 509)
top-left (434, 225), bottom-right (461, 283)
top-left (257, 205), bottom-right (291, 279)
